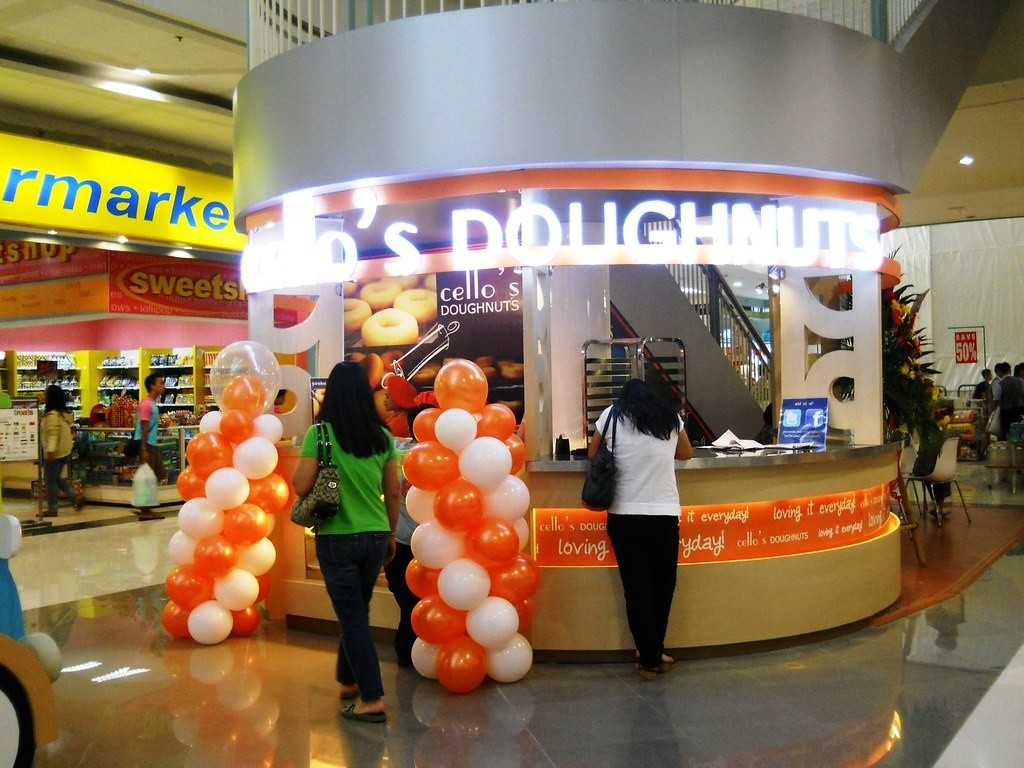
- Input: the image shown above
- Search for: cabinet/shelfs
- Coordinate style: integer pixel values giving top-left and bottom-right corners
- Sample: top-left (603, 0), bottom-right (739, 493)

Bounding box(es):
top-left (1, 346), bottom-right (247, 508)
top-left (937, 395), bottom-right (988, 462)
top-left (579, 334), bottom-right (688, 452)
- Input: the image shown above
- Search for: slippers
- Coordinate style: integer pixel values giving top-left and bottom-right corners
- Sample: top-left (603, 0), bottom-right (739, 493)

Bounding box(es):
top-left (137, 512), bottom-right (166, 520)
top-left (341, 703), bottom-right (387, 722)
top-left (36, 510), bottom-right (59, 517)
top-left (71, 495), bottom-right (85, 511)
top-left (338, 685), bottom-right (360, 701)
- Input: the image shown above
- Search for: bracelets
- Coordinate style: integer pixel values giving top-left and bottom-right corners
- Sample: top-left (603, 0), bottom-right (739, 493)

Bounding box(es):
top-left (388, 531), bottom-right (399, 536)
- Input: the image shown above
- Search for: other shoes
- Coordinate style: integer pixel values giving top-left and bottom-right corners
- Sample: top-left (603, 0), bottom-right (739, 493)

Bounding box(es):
top-left (929, 506), bottom-right (938, 518)
top-left (941, 505), bottom-right (952, 515)
top-left (639, 654), bottom-right (676, 672)
top-left (635, 659), bottom-right (658, 680)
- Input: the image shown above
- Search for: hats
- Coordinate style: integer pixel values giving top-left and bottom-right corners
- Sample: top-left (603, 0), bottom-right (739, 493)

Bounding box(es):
top-left (414, 391), bottom-right (443, 409)
top-left (384, 376), bottom-right (418, 408)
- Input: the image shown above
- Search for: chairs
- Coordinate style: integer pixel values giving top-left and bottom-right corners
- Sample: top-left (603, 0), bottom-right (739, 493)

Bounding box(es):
top-left (898, 436), bottom-right (973, 527)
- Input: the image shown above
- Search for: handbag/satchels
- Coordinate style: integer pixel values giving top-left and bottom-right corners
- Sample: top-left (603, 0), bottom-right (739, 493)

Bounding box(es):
top-left (582, 448), bottom-right (616, 511)
top-left (986, 406), bottom-right (1001, 436)
top-left (130, 462), bottom-right (159, 508)
top-left (290, 461), bottom-right (340, 525)
top-left (122, 438), bottom-right (143, 461)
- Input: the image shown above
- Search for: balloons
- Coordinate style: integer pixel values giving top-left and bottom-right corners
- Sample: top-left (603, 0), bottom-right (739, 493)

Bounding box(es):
top-left (160, 341), bottom-right (296, 646)
top-left (402, 359), bottom-right (543, 695)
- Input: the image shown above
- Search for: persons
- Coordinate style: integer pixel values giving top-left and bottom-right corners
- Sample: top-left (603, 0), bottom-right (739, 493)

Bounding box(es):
top-left (383, 374), bottom-right (418, 438)
top-left (290, 360), bottom-right (399, 723)
top-left (587, 378), bottom-right (694, 681)
top-left (382, 403), bottom-right (439, 667)
top-left (132, 372), bottom-right (167, 521)
top-left (37, 384), bottom-right (87, 518)
top-left (917, 361), bottom-right (1024, 518)
top-left (736, 362), bottom-right (773, 445)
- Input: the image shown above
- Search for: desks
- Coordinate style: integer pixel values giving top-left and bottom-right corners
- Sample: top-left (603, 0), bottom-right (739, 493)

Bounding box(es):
top-left (530, 440), bottom-right (906, 665)
